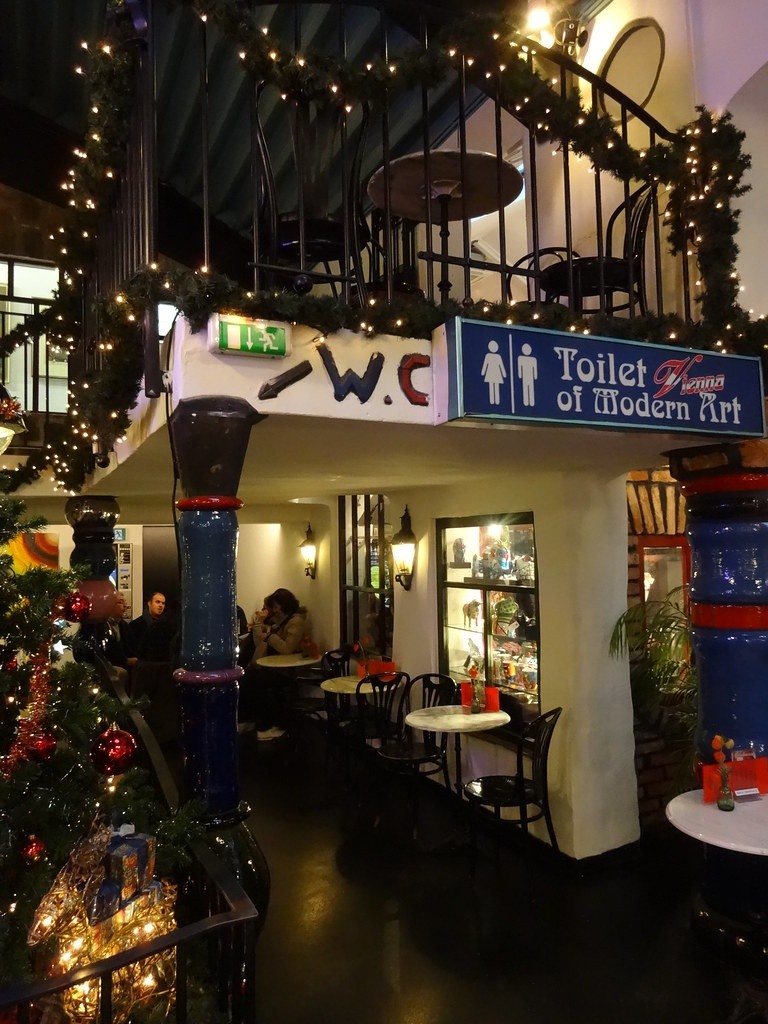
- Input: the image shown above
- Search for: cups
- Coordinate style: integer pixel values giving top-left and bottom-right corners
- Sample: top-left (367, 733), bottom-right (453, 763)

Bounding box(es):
top-left (495, 651), bottom-right (537, 689)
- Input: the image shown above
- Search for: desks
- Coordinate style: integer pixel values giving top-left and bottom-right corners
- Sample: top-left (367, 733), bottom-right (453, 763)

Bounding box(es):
top-left (367, 148), bottom-right (523, 305)
top-left (665, 789), bottom-right (768, 857)
top-left (404, 704), bottom-right (512, 852)
top-left (320, 675), bottom-right (403, 767)
top-left (254, 652), bottom-right (323, 728)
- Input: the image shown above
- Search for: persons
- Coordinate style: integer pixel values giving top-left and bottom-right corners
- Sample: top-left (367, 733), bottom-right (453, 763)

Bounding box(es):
top-left (112, 592), bottom-right (181, 742)
top-left (347, 585), bottom-right (394, 658)
top-left (237, 589), bottom-right (314, 742)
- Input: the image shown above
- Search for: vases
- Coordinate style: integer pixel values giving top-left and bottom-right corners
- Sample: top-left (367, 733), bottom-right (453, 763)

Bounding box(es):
top-left (715, 785), bottom-right (733, 811)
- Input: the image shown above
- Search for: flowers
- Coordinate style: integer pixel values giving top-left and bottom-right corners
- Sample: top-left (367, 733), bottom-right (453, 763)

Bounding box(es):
top-left (711, 736), bottom-right (734, 784)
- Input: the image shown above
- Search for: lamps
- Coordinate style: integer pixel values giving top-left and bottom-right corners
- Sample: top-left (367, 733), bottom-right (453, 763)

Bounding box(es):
top-left (297, 521), bottom-right (317, 579)
top-left (389, 503), bottom-right (417, 592)
top-left (527, 1), bottom-right (588, 57)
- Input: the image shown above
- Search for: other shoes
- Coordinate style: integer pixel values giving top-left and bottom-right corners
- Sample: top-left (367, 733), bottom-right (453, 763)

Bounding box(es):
top-left (256, 725), bottom-right (286, 741)
top-left (238, 720), bottom-right (257, 734)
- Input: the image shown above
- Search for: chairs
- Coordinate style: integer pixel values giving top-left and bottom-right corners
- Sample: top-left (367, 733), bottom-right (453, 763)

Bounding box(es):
top-left (372, 673), bottom-right (456, 840)
top-left (254, 78), bottom-right (374, 309)
top-left (285, 648), bottom-right (352, 753)
top-left (333, 671), bottom-right (410, 801)
top-left (540, 177), bottom-right (662, 322)
top-left (463, 705), bottom-right (565, 879)
top-left (506, 246), bottom-right (583, 310)
